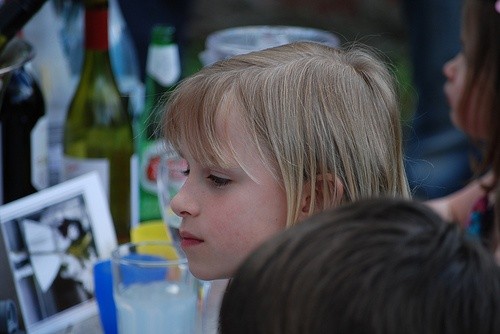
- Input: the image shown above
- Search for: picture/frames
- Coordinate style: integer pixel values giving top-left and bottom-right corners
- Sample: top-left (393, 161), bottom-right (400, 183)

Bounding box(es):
top-left (0, 172), bottom-right (119, 334)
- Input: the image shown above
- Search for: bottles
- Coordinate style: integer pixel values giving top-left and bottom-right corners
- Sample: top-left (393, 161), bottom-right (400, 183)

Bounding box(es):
top-left (60, 0), bottom-right (134, 252)
top-left (137, 25), bottom-right (205, 260)
top-left (0, 0), bottom-right (44, 204)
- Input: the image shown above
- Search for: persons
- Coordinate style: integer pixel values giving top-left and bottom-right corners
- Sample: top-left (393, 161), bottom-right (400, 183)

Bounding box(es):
top-left (217, 196), bottom-right (500, 334)
top-left (157, 42), bottom-right (414, 334)
top-left (420, 0), bottom-right (500, 267)
top-left (60, 218), bottom-right (100, 302)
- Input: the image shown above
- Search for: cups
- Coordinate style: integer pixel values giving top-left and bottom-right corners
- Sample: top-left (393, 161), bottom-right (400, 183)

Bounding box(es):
top-left (92, 256), bottom-right (168, 334)
top-left (110, 240), bottom-right (199, 334)
